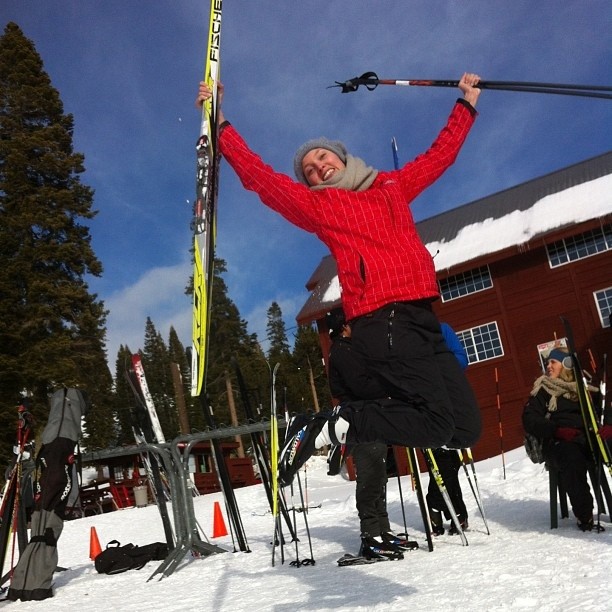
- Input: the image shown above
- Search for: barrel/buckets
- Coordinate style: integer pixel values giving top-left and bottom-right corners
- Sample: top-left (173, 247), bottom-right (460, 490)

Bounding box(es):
top-left (133, 486), bottom-right (148, 507)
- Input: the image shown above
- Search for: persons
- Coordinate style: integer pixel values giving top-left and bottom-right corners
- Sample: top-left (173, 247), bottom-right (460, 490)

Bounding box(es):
top-left (424, 445), bottom-right (468, 536)
top-left (195, 71), bottom-right (481, 486)
top-left (521, 346), bottom-right (600, 530)
top-left (324, 307), bottom-right (416, 562)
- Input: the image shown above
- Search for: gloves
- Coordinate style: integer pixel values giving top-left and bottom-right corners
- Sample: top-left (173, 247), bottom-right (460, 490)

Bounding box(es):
top-left (552, 426), bottom-right (580, 441)
top-left (597, 424), bottom-right (612, 440)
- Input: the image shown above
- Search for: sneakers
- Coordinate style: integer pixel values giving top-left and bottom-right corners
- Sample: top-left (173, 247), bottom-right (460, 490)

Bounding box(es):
top-left (359, 536), bottom-right (404, 561)
top-left (382, 531), bottom-right (419, 551)
top-left (277, 413), bottom-right (324, 487)
top-left (327, 405), bottom-right (355, 476)
top-left (429, 508), bottom-right (446, 536)
top-left (449, 514), bottom-right (468, 535)
top-left (577, 519), bottom-right (594, 531)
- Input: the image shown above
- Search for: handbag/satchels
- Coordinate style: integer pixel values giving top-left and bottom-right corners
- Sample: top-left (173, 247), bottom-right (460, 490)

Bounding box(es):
top-left (94, 540), bottom-right (139, 575)
top-left (523, 432), bottom-right (545, 462)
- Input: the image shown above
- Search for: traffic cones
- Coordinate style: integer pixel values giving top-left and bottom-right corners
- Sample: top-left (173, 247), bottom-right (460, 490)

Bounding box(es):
top-left (89, 526), bottom-right (102, 560)
top-left (209, 501), bottom-right (229, 538)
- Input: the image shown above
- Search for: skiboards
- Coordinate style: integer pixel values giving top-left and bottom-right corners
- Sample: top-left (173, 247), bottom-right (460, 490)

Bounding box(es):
top-left (263, 359), bottom-right (279, 518)
top-left (190, 1), bottom-right (223, 398)
top-left (405, 448), bottom-right (469, 553)
top-left (565, 338), bottom-right (612, 525)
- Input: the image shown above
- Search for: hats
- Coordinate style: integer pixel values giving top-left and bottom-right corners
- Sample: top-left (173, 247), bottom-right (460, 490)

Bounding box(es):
top-left (294, 137), bottom-right (348, 185)
top-left (325, 308), bottom-right (348, 340)
top-left (547, 348), bottom-right (570, 362)
